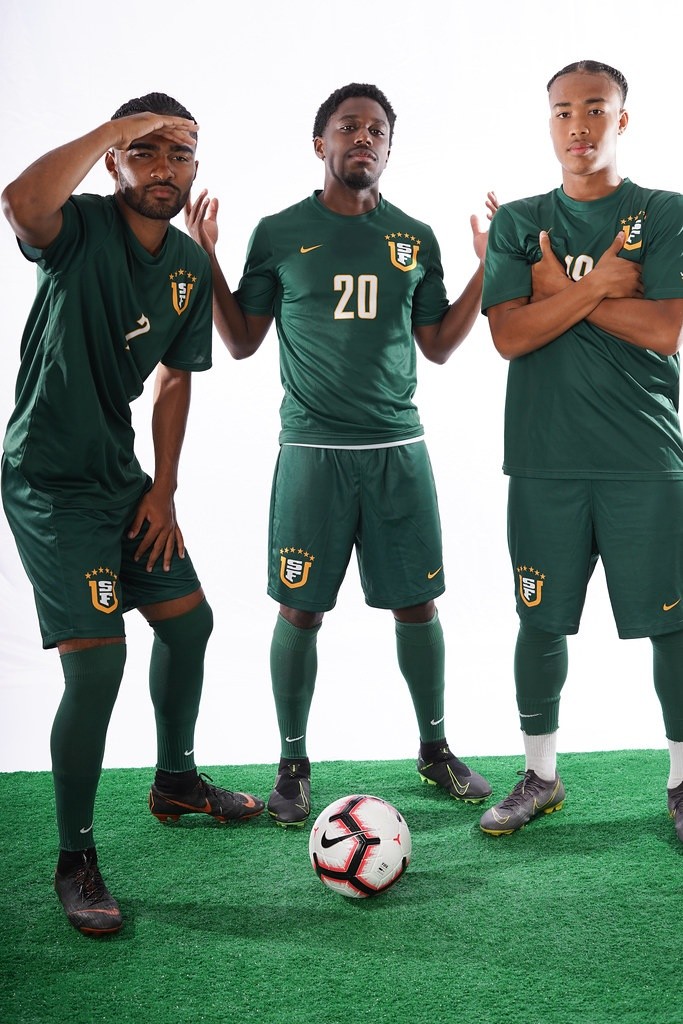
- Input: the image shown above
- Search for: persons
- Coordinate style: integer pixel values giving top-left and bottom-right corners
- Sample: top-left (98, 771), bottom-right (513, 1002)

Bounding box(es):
top-left (182, 83), bottom-right (498, 826)
top-left (0, 90), bottom-right (265, 933)
top-left (477, 61), bottom-right (682, 848)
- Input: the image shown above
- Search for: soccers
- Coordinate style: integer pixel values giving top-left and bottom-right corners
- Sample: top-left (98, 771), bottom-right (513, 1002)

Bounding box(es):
top-left (309, 794), bottom-right (411, 898)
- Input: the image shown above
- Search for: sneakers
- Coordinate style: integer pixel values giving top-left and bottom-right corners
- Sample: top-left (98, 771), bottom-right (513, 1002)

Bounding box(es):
top-left (416, 745), bottom-right (492, 805)
top-left (478, 768), bottom-right (567, 837)
top-left (266, 756), bottom-right (311, 829)
top-left (148, 766), bottom-right (265, 825)
top-left (54, 846), bottom-right (124, 935)
top-left (665, 780), bottom-right (683, 843)
